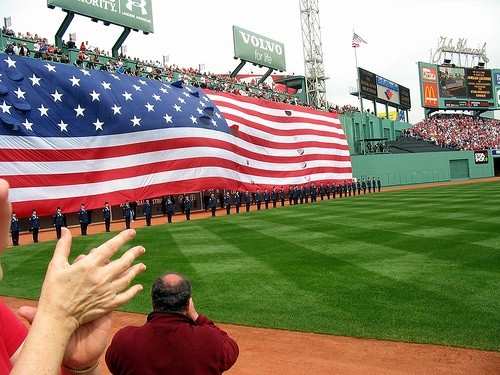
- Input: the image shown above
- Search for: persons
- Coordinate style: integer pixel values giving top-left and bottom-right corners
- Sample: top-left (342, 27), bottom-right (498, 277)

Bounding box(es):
top-left (319, 177), bottom-right (381, 200)
top-left (105, 272), bottom-right (240, 375)
top-left (329, 104), bottom-right (358, 114)
top-left (142, 59), bottom-right (316, 108)
top-left (53, 208), bottom-right (67, 239)
top-left (11, 212), bottom-right (21, 246)
top-left (0, 226), bottom-right (146, 375)
top-left (103, 202), bottom-right (111, 232)
top-left (234, 182), bottom-right (317, 213)
top-left (122, 199), bottom-right (154, 229)
top-left (162, 190), bottom-right (231, 223)
top-left (401, 115), bottom-right (500, 151)
top-left (78, 203), bottom-right (91, 235)
top-left (2, 26), bottom-right (70, 63)
top-left (63, 37), bottom-right (143, 77)
top-left (439, 72), bottom-right (466, 97)
top-left (367, 141), bottom-right (388, 152)
top-left (30, 210), bottom-right (40, 243)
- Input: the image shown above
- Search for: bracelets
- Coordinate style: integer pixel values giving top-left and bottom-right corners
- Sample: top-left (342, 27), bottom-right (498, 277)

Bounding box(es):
top-left (62, 359), bottom-right (100, 373)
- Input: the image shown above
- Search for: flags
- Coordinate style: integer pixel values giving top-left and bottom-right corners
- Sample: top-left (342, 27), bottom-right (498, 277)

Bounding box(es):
top-left (352, 33), bottom-right (367, 47)
